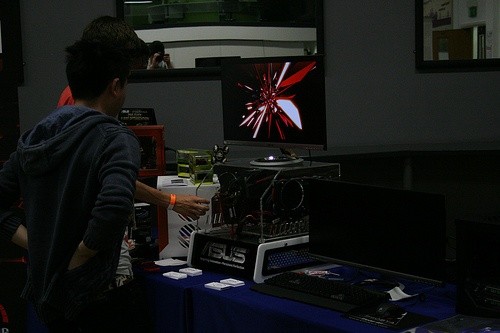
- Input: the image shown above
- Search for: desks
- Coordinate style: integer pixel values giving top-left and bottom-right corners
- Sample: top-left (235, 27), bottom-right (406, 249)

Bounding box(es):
top-left (24, 255), bottom-right (459, 333)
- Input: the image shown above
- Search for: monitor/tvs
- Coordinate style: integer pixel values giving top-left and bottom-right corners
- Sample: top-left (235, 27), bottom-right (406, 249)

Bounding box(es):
top-left (306, 175), bottom-right (450, 292)
top-left (220, 52), bottom-right (328, 167)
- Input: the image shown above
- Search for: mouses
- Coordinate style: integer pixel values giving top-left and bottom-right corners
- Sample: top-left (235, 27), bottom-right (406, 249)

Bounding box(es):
top-left (374, 303), bottom-right (399, 316)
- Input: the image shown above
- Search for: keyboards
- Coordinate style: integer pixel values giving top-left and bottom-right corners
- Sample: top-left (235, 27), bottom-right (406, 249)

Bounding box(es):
top-left (264, 271), bottom-right (390, 305)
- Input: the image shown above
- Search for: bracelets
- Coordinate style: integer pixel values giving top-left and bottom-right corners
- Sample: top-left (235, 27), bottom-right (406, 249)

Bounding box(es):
top-left (168, 193), bottom-right (176, 210)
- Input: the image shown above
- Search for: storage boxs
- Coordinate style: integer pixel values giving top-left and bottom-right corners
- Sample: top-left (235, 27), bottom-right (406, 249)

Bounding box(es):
top-left (157, 174), bottom-right (220, 261)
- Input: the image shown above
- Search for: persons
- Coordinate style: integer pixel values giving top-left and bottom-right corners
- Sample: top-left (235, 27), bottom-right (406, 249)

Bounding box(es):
top-left (0, 36), bottom-right (158, 333)
top-left (146, 41), bottom-right (173, 68)
top-left (56, 14), bottom-right (210, 333)
